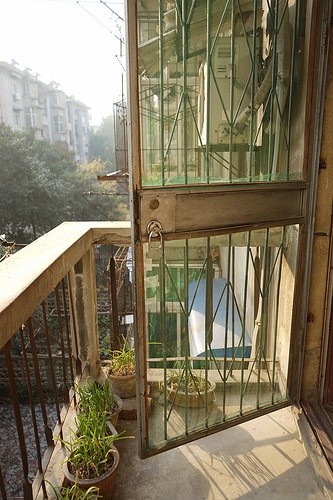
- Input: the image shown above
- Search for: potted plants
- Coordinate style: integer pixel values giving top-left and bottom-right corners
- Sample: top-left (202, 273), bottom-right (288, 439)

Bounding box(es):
top-left (146, 343), bottom-right (216, 409)
top-left (68, 375), bottom-right (122, 428)
top-left (97, 332), bottom-right (136, 398)
top-left (50, 398), bottom-right (136, 500)
